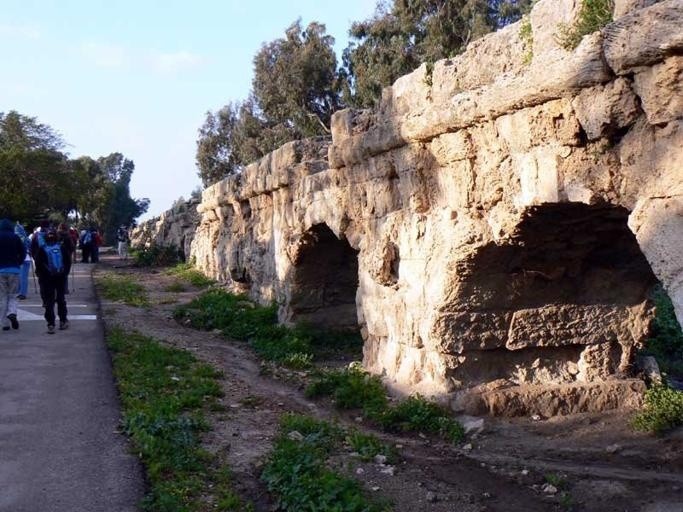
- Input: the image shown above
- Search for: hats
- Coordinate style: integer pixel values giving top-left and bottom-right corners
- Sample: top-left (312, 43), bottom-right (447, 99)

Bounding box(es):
top-left (15, 224), bottom-right (24, 236)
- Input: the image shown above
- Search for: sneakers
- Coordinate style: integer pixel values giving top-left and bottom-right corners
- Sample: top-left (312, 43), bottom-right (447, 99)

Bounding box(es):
top-left (17, 295), bottom-right (25, 299)
top-left (60, 320), bottom-right (69, 329)
top-left (48, 326), bottom-right (55, 333)
top-left (3, 314), bottom-right (18, 330)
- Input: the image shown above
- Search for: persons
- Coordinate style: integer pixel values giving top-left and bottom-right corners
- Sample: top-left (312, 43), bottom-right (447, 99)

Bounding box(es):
top-left (115, 224), bottom-right (131, 260)
top-left (0, 212), bottom-right (106, 334)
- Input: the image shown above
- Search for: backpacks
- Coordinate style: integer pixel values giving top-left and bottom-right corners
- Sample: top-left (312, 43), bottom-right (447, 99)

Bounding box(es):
top-left (38, 232), bottom-right (64, 273)
